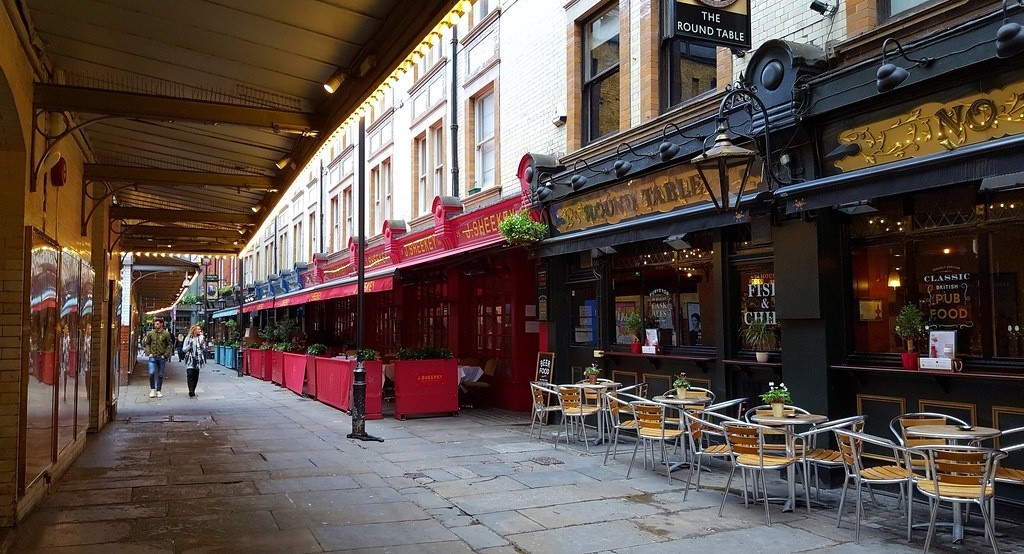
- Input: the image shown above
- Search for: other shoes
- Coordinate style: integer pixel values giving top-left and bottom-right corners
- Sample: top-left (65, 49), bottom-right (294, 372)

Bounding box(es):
top-left (189, 391), bottom-right (195, 397)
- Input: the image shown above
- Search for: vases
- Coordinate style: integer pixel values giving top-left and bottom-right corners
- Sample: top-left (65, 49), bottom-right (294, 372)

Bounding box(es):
top-left (677, 387), bottom-right (686, 399)
top-left (770, 402), bottom-right (784, 417)
top-left (589, 374), bottom-right (597, 384)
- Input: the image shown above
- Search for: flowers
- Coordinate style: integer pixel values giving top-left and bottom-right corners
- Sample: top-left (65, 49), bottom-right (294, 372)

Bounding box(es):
top-left (583, 361), bottom-right (602, 376)
top-left (673, 372), bottom-right (691, 390)
top-left (759, 382), bottom-right (793, 404)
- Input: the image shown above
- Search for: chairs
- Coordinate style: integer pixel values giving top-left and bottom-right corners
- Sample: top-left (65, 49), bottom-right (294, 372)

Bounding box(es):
top-left (345, 349), bottom-right (499, 411)
top-left (530, 379), bottom-right (1024, 554)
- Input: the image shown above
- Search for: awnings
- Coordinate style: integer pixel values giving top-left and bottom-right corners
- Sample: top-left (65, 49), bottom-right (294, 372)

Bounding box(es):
top-left (212, 235), bottom-right (534, 320)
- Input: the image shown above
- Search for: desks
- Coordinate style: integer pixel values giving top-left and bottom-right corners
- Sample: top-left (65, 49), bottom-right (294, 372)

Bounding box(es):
top-left (458, 365), bottom-right (484, 409)
top-left (750, 413), bottom-right (835, 513)
top-left (652, 396), bottom-right (712, 473)
top-left (382, 364), bottom-right (394, 402)
top-left (559, 383), bottom-right (626, 446)
top-left (904, 424), bottom-right (1006, 544)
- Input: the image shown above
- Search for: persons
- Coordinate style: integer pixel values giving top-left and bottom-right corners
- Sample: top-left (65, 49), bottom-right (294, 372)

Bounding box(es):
top-left (137, 328), bottom-right (208, 362)
top-left (145, 318), bottom-right (171, 398)
top-left (84, 324), bottom-right (92, 399)
top-left (182, 325), bottom-right (206, 398)
top-left (691, 313), bottom-right (701, 332)
top-left (61, 324), bottom-right (70, 404)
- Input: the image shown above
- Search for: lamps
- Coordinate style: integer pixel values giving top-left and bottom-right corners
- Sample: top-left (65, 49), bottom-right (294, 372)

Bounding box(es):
top-left (995, 0), bottom-right (1024, 58)
top-left (275, 153), bottom-right (293, 169)
top-left (614, 142), bottom-right (652, 179)
top-left (972, 170), bottom-right (1024, 192)
top-left (251, 202), bottom-right (262, 212)
top-left (810, 0), bottom-right (837, 17)
top-left (659, 123), bottom-right (704, 164)
top-left (691, 70), bottom-right (790, 216)
top-left (832, 199), bottom-right (881, 215)
top-left (536, 172), bottom-right (572, 202)
top-left (237, 225), bottom-right (248, 234)
top-left (876, 38), bottom-right (929, 94)
top-left (323, 66), bottom-right (352, 94)
top-left (596, 245), bottom-right (622, 256)
top-left (662, 232), bottom-right (696, 251)
top-left (571, 158), bottom-right (605, 192)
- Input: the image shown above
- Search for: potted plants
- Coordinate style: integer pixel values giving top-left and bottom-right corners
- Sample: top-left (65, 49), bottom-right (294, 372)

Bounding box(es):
top-left (224, 319), bottom-right (240, 350)
top-left (623, 312), bottom-right (643, 354)
top-left (895, 302), bottom-right (927, 370)
top-left (738, 318), bottom-right (780, 363)
top-left (497, 207), bottom-right (548, 248)
top-left (218, 285), bottom-right (233, 297)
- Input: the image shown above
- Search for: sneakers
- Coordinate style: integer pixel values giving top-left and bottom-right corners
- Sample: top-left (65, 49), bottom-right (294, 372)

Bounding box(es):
top-left (156, 390), bottom-right (163, 398)
top-left (150, 388), bottom-right (156, 397)
